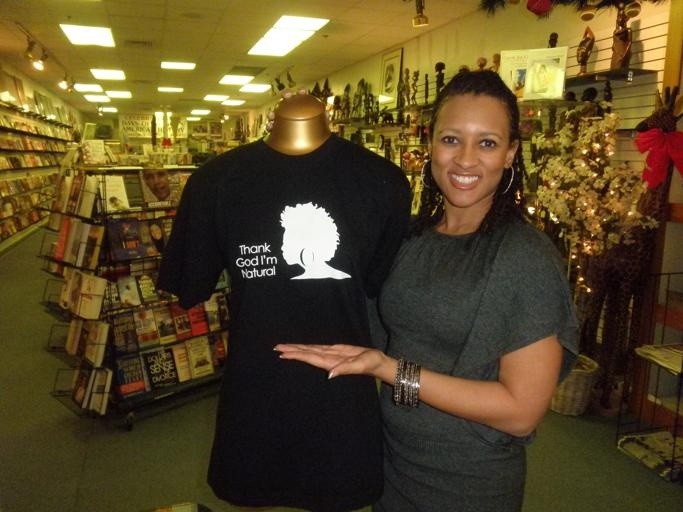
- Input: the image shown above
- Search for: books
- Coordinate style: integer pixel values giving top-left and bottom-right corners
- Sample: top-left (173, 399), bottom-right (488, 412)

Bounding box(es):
top-left (1, 114), bottom-right (72, 241)
top-left (48, 169), bottom-right (229, 416)
top-left (61, 123), bottom-right (205, 166)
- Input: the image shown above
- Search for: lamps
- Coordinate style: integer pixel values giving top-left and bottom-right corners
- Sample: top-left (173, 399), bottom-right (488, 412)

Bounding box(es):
top-left (407, 0), bottom-right (429, 29)
top-left (21, 35), bottom-right (73, 95)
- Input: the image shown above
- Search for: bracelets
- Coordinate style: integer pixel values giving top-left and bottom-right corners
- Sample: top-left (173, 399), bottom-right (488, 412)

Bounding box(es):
top-left (394, 355), bottom-right (421, 408)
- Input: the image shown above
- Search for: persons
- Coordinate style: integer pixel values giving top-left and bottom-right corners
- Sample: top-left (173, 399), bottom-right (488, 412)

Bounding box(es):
top-left (266, 69), bottom-right (579, 512)
top-left (157, 88), bottom-right (412, 512)
top-left (143, 169), bottom-right (170, 200)
top-left (150, 224), bottom-right (162, 240)
top-left (74, 377), bottom-right (86, 403)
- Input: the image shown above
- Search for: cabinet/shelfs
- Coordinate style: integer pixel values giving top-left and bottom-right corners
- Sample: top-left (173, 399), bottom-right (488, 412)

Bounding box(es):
top-left (1, 104), bottom-right (81, 248)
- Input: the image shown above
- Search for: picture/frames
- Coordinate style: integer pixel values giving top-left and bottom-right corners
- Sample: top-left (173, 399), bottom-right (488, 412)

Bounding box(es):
top-left (500, 47), bottom-right (566, 102)
top-left (378, 47), bottom-right (402, 113)
